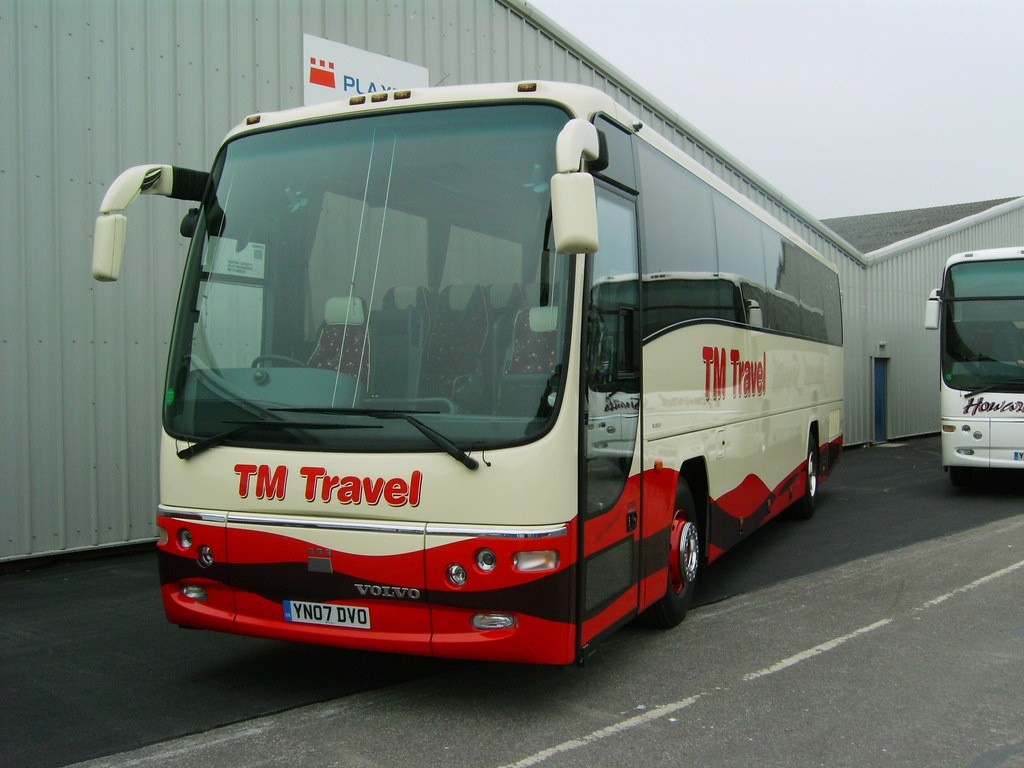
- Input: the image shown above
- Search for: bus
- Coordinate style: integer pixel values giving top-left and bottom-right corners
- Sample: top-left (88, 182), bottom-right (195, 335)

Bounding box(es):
top-left (924, 246), bottom-right (1024, 488)
top-left (91, 83), bottom-right (847, 667)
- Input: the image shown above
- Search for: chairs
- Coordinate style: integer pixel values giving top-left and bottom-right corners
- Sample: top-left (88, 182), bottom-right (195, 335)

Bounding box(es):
top-left (307, 283), bottom-right (560, 417)
